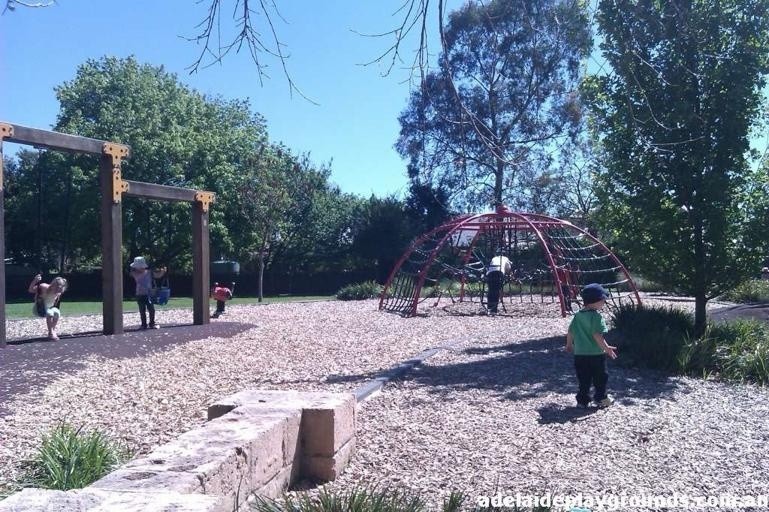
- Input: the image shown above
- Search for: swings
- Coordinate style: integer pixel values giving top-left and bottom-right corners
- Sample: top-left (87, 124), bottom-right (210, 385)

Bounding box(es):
top-left (37, 146), bottom-right (73, 317)
top-left (146, 198), bottom-right (172, 305)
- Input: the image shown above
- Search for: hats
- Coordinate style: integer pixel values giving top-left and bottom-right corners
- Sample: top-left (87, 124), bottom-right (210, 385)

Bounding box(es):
top-left (129, 256), bottom-right (149, 269)
top-left (582, 282), bottom-right (612, 307)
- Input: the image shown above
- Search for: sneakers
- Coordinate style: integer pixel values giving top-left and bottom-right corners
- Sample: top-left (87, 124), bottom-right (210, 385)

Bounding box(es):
top-left (576, 397), bottom-right (611, 410)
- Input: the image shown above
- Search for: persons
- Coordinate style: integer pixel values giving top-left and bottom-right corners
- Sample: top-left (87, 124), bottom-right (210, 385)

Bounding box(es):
top-left (565, 282), bottom-right (618, 409)
top-left (129, 255), bottom-right (168, 329)
top-left (28, 273), bottom-right (68, 343)
top-left (209, 280), bottom-right (237, 315)
top-left (485, 247), bottom-right (514, 315)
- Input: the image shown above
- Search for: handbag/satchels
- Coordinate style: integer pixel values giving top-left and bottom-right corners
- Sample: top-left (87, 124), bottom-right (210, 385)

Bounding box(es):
top-left (149, 273), bottom-right (171, 306)
